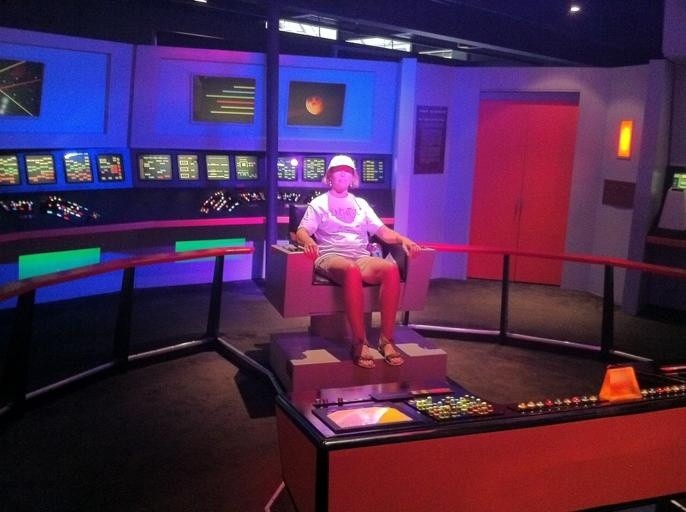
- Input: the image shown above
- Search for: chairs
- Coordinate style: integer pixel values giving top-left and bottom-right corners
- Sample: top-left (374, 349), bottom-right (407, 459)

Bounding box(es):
top-left (267, 203), bottom-right (450, 394)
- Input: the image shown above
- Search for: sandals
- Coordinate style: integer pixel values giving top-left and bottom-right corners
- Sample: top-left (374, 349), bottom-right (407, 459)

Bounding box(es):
top-left (376, 337), bottom-right (404, 366)
top-left (350, 339), bottom-right (376, 369)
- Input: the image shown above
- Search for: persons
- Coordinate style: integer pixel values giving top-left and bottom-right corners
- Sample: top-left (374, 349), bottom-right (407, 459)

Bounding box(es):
top-left (295, 154), bottom-right (422, 369)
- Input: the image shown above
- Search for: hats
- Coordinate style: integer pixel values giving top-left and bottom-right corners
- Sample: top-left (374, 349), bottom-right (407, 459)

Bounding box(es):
top-left (322, 155), bottom-right (359, 190)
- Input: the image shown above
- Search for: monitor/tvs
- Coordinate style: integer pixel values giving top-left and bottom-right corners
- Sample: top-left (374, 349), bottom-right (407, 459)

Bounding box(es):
top-left (63, 151), bottom-right (94, 183)
top-left (673, 172), bottom-right (686, 191)
top-left (204, 152), bottom-right (231, 181)
top-left (278, 155), bottom-right (299, 182)
top-left (0, 153), bottom-right (22, 187)
top-left (134, 151), bottom-right (174, 182)
top-left (176, 154), bottom-right (201, 181)
top-left (97, 153), bottom-right (123, 183)
top-left (361, 156), bottom-right (386, 184)
top-left (24, 153), bottom-right (59, 185)
top-left (234, 155), bottom-right (259, 180)
top-left (302, 156), bottom-right (327, 182)
